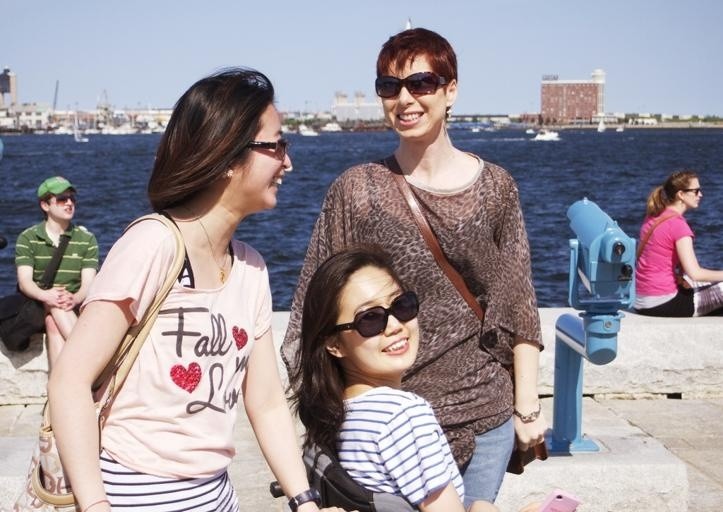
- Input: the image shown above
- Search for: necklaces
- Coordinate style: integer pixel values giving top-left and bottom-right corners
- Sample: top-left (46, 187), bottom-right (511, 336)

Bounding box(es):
top-left (185, 205), bottom-right (230, 285)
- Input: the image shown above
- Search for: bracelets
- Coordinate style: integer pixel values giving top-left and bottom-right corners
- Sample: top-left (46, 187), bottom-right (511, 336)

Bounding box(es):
top-left (514, 400), bottom-right (544, 427)
top-left (288, 486), bottom-right (325, 512)
top-left (80, 499), bottom-right (111, 512)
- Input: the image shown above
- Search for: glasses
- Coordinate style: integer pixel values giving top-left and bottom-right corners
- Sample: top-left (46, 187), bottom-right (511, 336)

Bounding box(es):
top-left (54, 194), bottom-right (78, 203)
top-left (244, 137), bottom-right (291, 160)
top-left (373, 73), bottom-right (447, 98)
top-left (325, 289), bottom-right (421, 338)
top-left (682, 187), bottom-right (703, 196)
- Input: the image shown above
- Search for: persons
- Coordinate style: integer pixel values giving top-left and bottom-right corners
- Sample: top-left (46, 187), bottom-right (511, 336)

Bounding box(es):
top-left (42, 67), bottom-right (362, 512)
top-left (279, 28), bottom-right (549, 512)
top-left (284, 243), bottom-right (579, 511)
top-left (13, 175), bottom-right (99, 378)
top-left (628, 169), bottom-right (723, 318)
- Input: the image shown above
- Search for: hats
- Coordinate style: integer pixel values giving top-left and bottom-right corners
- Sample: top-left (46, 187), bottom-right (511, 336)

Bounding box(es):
top-left (36, 175), bottom-right (76, 198)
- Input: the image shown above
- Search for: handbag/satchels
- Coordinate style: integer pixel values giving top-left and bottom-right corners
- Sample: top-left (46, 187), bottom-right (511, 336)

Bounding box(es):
top-left (506, 437), bottom-right (547, 474)
top-left (11, 397), bottom-right (104, 511)
top-left (0, 283), bottom-right (52, 351)
